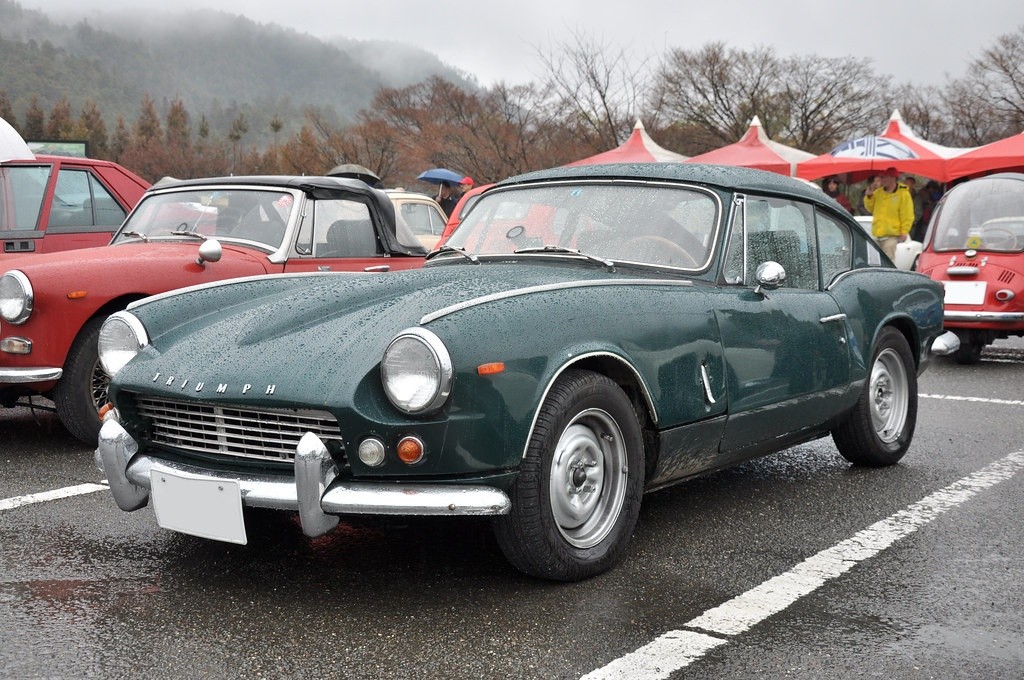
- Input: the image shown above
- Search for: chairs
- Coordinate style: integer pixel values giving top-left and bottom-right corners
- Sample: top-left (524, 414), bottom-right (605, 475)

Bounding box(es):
top-left (721, 230), bottom-right (803, 288)
top-left (317, 219), bottom-right (377, 258)
top-left (68, 197), bottom-right (125, 225)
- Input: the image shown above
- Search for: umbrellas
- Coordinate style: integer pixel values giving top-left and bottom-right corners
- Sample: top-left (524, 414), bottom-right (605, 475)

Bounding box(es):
top-left (327, 164), bottom-right (380, 187)
top-left (417, 168), bottom-right (463, 196)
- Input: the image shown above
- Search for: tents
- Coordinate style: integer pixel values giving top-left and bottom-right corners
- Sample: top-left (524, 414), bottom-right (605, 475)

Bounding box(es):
top-left (796, 108), bottom-right (1024, 193)
top-left (567, 120), bottom-right (691, 163)
top-left (684, 115), bottom-right (819, 178)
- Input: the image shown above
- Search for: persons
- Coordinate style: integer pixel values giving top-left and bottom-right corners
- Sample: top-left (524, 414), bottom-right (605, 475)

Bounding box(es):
top-left (432, 181), bottom-right (460, 219)
top-left (858, 168), bottom-right (970, 261)
top-left (459, 176), bottom-right (475, 200)
top-left (824, 174), bottom-right (851, 212)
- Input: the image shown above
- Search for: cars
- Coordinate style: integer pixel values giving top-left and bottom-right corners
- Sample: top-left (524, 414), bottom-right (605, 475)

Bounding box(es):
top-left (914, 171), bottom-right (1024, 364)
top-left (92, 162), bottom-right (946, 587)
top-left (850, 215), bottom-right (922, 271)
top-left (0, 155), bottom-right (155, 261)
top-left (0, 173), bottom-right (434, 446)
top-left (379, 190), bottom-right (449, 253)
